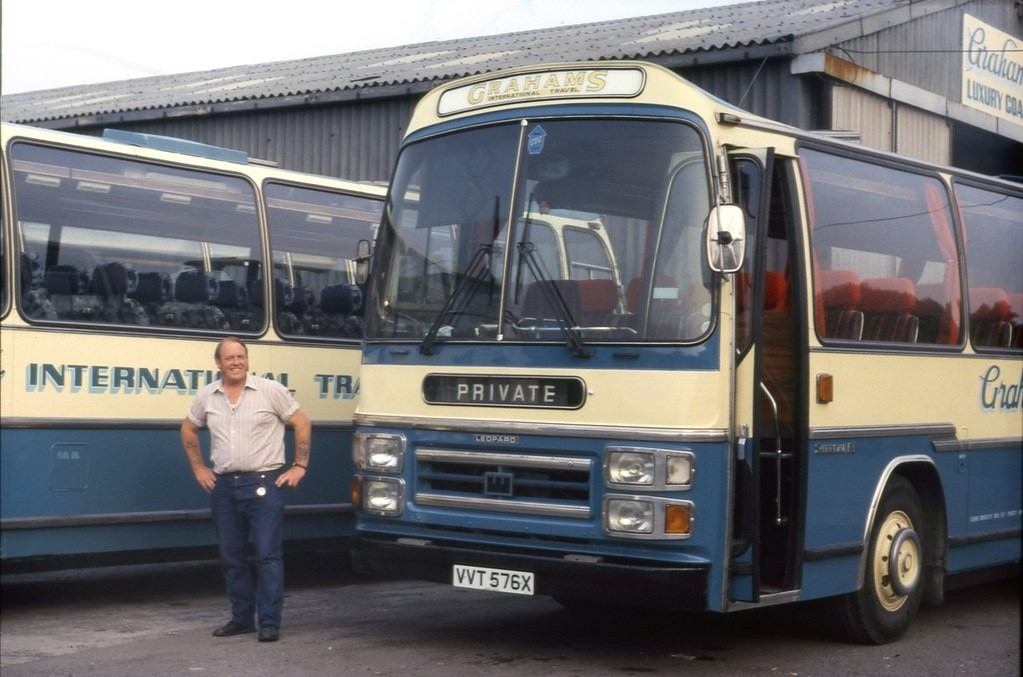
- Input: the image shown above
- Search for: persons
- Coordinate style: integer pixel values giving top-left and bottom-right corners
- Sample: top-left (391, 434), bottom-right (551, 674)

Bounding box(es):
top-left (181, 336), bottom-right (311, 642)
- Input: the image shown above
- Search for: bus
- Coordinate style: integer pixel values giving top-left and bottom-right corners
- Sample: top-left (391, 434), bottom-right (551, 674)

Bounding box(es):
top-left (0, 123), bottom-right (634, 589)
top-left (347, 59), bottom-right (1023, 644)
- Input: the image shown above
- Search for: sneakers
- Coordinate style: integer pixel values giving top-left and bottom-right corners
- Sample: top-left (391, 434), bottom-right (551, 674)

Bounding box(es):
top-left (213, 620), bottom-right (255, 636)
top-left (258, 624), bottom-right (279, 641)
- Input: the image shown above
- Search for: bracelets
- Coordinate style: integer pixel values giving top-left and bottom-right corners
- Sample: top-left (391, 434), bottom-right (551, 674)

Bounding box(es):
top-left (292, 462), bottom-right (308, 471)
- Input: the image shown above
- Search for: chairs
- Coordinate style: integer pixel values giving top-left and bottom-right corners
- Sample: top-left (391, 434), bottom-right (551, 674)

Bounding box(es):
top-left (518, 269), bottom-right (1022, 349)
top-left (0, 252), bottom-right (365, 340)
